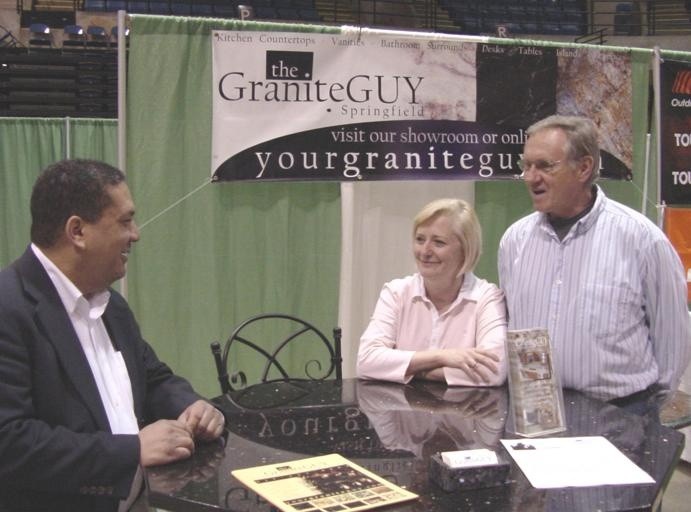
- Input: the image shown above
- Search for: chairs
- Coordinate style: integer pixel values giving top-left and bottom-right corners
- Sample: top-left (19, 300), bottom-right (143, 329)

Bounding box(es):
top-left (442, 0), bottom-right (591, 36)
top-left (29, 0), bottom-right (319, 52)
top-left (212, 314), bottom-right (343, 395)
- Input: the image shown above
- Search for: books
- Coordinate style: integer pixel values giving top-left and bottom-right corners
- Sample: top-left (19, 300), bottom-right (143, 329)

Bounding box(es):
top-left (230, 452), bottom-right (420, 512)
top-left (505, 374), bottom-right (568, 440)
top-left (503, 327), bottom-right (556, 384)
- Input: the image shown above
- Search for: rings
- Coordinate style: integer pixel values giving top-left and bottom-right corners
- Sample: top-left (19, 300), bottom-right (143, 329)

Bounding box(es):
top-left (471, 361), bottom-right (479, 368)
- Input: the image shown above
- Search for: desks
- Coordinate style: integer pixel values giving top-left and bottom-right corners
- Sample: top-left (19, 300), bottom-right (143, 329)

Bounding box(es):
top-left (145, 377), bottom-right (685, 512)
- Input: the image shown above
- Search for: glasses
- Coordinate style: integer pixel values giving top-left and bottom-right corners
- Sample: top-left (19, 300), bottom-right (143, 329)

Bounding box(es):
top-left (516, 157), bottom-right (566, 176)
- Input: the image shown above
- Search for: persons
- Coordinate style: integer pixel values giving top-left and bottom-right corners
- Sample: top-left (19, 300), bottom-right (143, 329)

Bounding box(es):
top-left (0, 159), bottom-right (226, 512)
top-left (501, 389), bottom-right (659, 512)
top-left (352, 379), bottom-right (509, 512)
top-left (144, 432), bottom-right (226, 499)
top-left (494, 116), bottom-right (691, 426)
top-left (355, 199), bottom-right (509, 389)
